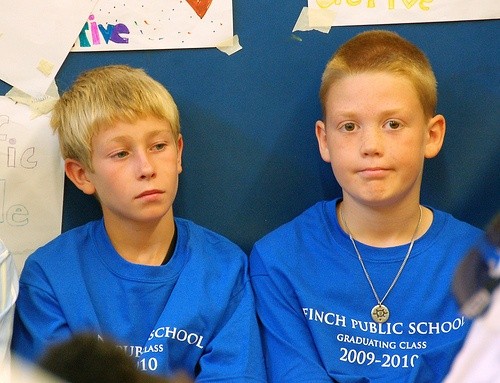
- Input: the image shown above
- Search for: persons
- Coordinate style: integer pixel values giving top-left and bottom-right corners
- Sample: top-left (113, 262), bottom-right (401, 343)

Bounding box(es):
top-left (10, 65), bottom-right (268, 383)
top-left (249, 30), bottom-right (500, 383)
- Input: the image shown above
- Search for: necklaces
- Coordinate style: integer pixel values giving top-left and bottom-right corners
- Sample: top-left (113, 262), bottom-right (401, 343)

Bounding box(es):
top-left (340, 201), bottom-right (422, 324)
